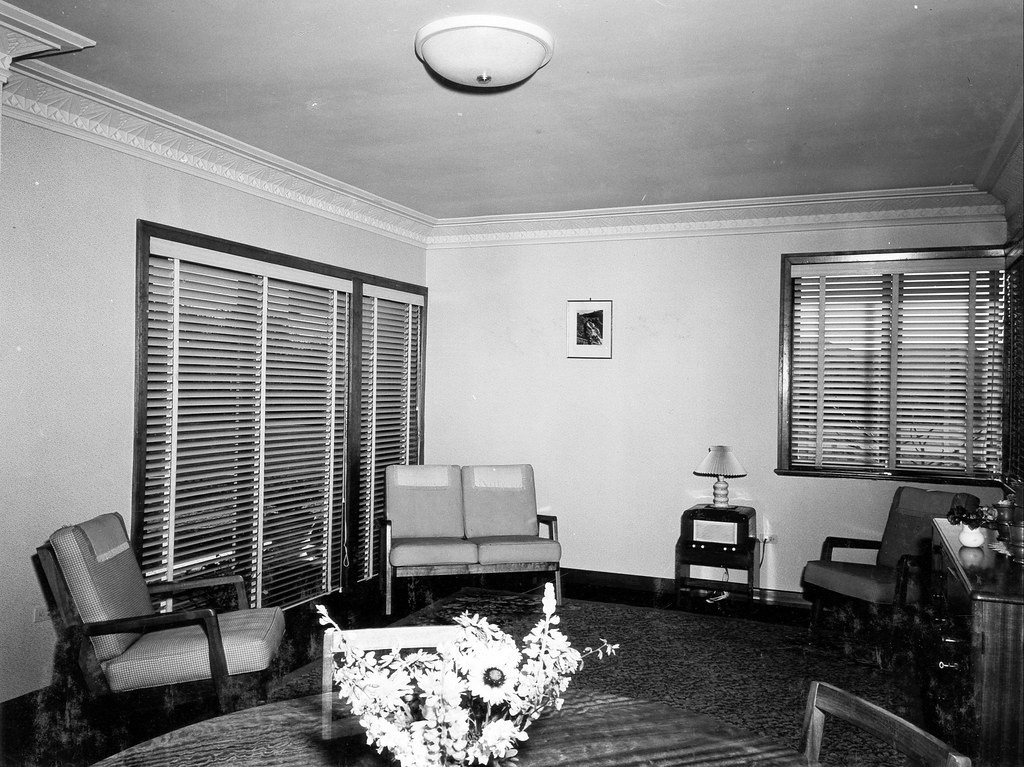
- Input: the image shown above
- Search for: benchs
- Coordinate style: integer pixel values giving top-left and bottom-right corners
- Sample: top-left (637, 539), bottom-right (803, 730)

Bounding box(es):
top-left (384, 463), bottom-right (561, 616)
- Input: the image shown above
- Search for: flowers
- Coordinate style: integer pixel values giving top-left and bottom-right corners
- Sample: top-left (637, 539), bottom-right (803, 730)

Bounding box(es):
top-left (315, 582), bottom-right (620, 767)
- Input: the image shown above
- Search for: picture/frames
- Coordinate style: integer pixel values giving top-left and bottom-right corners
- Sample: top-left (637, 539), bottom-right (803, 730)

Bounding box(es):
top-left (566, 299), bottom-right (613, 359)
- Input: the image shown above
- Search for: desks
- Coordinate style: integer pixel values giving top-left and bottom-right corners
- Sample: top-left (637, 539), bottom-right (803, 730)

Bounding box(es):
top-left (99, 692), bottom-right (818, 767)
top-left (675, 505), bottom-right (761, 601)
top-left (928, 517), bottom-right (1024, 767)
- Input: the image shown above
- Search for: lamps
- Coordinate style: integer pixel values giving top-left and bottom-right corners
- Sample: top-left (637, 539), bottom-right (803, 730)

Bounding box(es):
top-left (414, 15), bottom-right (554, 88)
top-left (695, 445), bottom-right (747, 509)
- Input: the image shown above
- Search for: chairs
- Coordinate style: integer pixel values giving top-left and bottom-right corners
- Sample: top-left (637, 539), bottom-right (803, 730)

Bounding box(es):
top-left (799, 683), bottom-right (973, 767)
top-left (37, 511), bottom-right (286, 719)
top-left (799, 486), bottom-right (980, 663)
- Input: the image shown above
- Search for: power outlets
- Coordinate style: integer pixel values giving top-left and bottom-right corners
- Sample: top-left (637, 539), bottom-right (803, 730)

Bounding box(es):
top-left (763, 534), bottom-right (778, 545)
